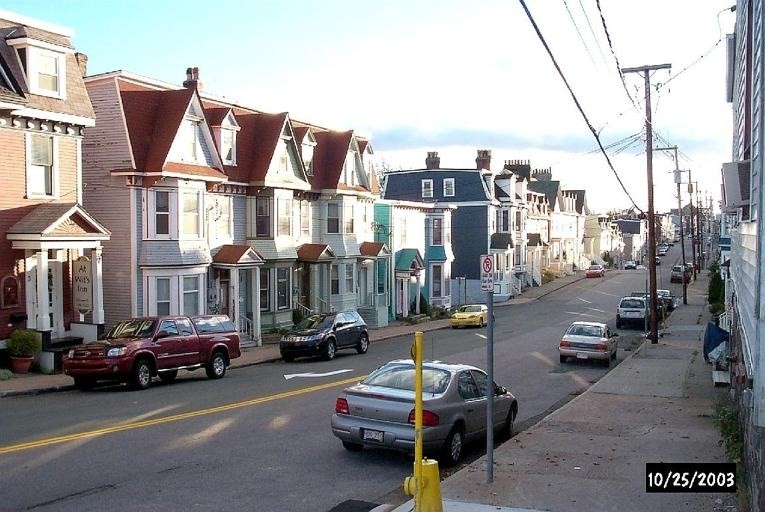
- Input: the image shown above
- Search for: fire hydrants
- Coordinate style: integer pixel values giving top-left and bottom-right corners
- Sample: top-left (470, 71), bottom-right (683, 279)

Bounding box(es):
top-left (402, 457), bottom-right (444, 512)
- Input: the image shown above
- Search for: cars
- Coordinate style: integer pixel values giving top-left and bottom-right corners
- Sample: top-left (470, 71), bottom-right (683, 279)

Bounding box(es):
top-left (623, 260), bottom-right (637, 270)
top-left (686, 261), bottom-right (694, 269)
top-left (330, 358), bottom-right (522, 467)
top-left (688, 232), bottom-right (701, 244)
top-left (450, 303), bottom-right (495, 330)
top-left (275, 308), bottom-right (371, 363)
top-left (557, 319), bottom-right (620, 368)
top-left (616, 289), bottom-right (676, 331)
top-left (655, 234), bottom-right (685, 265)
top-left (584, 264), bottom-right (605, 278)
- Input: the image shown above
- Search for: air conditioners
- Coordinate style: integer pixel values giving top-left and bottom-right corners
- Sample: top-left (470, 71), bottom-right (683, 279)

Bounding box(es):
top-left (722, 159), bottom-right (751, 210)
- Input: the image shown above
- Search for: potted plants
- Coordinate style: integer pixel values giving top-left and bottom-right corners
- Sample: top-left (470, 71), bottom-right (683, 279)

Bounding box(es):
top-left (4, 327), bottom-right (42, 375)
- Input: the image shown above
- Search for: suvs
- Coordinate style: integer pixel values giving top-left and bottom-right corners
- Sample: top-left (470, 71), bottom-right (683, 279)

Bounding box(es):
top-left (669, 262), bottom-right (688, 282)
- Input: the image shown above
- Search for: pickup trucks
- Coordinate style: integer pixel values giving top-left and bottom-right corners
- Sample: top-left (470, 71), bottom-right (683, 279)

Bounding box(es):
top-left (60, 312), bottom-right (243, 392)
